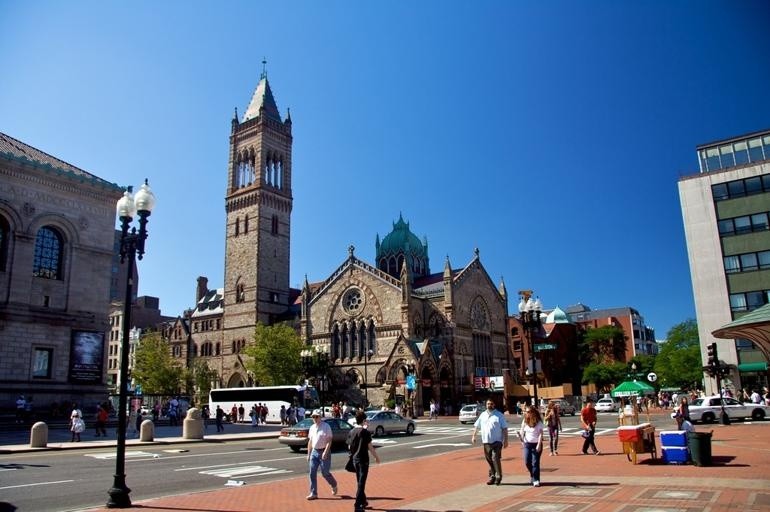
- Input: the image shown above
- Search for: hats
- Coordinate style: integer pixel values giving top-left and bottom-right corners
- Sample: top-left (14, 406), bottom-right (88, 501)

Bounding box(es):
top-left (310, 409), bottom-right (323, 417)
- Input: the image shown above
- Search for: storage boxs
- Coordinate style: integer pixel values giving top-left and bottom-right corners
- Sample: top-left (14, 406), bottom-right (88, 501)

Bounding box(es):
top-left (659, 430), bottom-right (689, 464)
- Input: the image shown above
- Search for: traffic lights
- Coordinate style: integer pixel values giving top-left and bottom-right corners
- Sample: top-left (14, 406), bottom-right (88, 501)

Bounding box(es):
top-left (707, 343), bottom-right (717, 366)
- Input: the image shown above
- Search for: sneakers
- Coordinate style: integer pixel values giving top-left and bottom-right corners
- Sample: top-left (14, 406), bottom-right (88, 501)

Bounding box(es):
top-left (332, 486), bottom-right (337, 495)
top-left (595, 451), bottom-right (600, 455)
top-left (495, 479), bottom-right (500, 484)
top-left (307, 494), bottom-right (317, 500)
top-left (360, 502), bottom-right (368, 507)
top-left (534, 480), bottom-right (540, 487)
top-left (529, 476), bottom-right (533, 483)
top-left (583, 449), bottom-right (587, 455)
top-left (555, 452), bottom-right (558, 456)
top-left (487, 479), bottom-right (494, 485)
top-left (550, 453), bottom-right (554, 456)
top-left (355, 509), bottom-right (364, 512)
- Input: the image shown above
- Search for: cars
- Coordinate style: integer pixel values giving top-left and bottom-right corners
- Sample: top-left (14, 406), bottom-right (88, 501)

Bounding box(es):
top-left (458, 404), bottom-right (485, 424)
top-left (278, 417), bottom-right (355, 452)
top-left (318, 406), bottom-right (417, 437)
top-left (594, 398), bottom-right (620, 413)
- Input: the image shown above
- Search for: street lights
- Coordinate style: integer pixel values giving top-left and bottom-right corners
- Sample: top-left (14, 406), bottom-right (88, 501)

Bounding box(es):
top-left (364, 347), bottom-right (374, 406)
top-left (517, 296), bottom-right (543, 414)
top-left (108, 176), bottom-right (155, 508)
top-left (631, 363), bottom-right (636, 382)
top-left (525, 367), bottom-right (531, 408)
top-left (405, 359), bottom-right (418, 419)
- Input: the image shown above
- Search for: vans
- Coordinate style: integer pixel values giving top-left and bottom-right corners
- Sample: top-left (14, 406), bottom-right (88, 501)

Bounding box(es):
top-left (670, 396), bottom-right (770, 424)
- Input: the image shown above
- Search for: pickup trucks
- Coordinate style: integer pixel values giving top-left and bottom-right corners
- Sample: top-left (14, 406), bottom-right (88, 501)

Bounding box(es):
top-left (541, 398), bottom-right (576, 416)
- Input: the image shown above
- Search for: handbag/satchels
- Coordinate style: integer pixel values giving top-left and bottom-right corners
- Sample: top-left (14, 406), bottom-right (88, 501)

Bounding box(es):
top-left (345, 455), bottom-right (356, 473)
top-left (581, 431), bottom-right (590, 437)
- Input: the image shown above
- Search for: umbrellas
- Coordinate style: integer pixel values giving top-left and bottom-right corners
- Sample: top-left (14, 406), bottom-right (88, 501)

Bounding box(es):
top-left (610, 378), bottom-right (655, 397)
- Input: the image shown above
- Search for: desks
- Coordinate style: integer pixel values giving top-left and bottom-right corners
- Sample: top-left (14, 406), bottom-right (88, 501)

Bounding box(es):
top-left (616, 422), bottom-right (658, 465)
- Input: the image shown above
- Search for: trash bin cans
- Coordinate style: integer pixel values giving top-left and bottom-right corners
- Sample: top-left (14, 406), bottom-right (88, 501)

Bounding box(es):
top-left (688, 432), bottom-right (712, 466)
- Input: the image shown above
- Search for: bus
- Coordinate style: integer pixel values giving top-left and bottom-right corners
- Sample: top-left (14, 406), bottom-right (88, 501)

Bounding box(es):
top-left (209, 383), bottom-right (322, 423)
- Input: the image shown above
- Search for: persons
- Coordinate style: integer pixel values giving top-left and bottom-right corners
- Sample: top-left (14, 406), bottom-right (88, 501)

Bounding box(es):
top-left (303, 406), bottom-right (338, 500)
top-left (676, 395), bottom-right (691, 422)
top-left (368, 398), bottom-right (440, 420)
top-left (443, 397), bottom-right (452, 416)
top-left (94, 405), bottom-right (109, 437)
top-left (471, 397), bottom-right (509, 486)
top-left (346, 410), bottom-right (379, 511)
top-left (136, 404), bottom-right (178, 435)
top-left (15, 394), bottom-right (35, 424)
top-left (204, 403), bottom-right (269, 433)
top-left (628, 383), bottom-right (770, 413)
top-left (519, 404), bottom-right (545, 487)
top-left (578, 398), bottom-right (601, 456)
top-left (71, 402), bottom-right (83, 442)
top-left (280, 404), bottom-right (303, 427)
top-left (336, 400), bottom-right (347, 422)
top-left (543, 400), bottom-right (562, 457)
